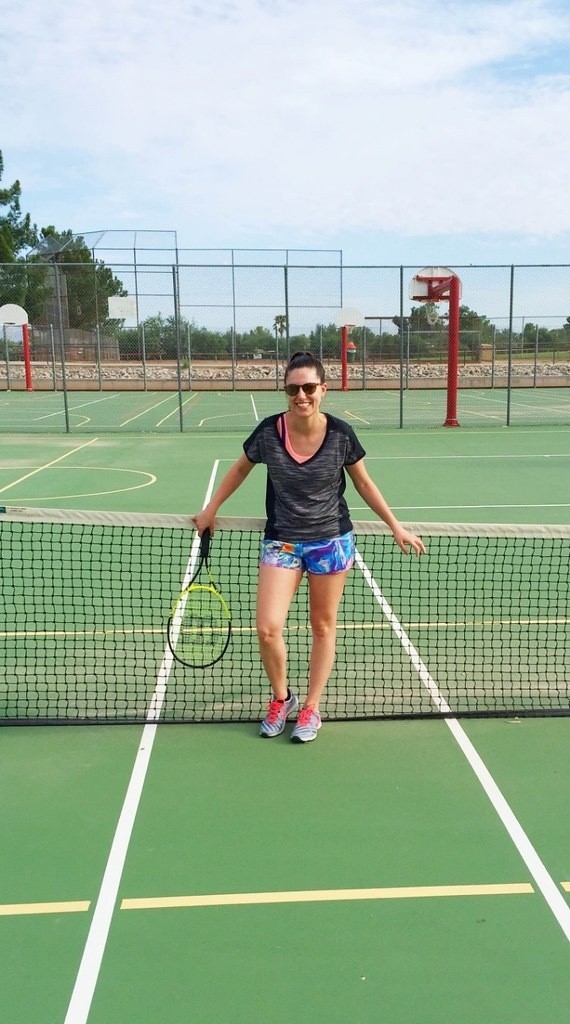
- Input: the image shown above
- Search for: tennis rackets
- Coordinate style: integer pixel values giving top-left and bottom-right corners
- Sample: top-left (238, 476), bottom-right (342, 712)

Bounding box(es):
top-left (166, 527), bottom-right (234, 670)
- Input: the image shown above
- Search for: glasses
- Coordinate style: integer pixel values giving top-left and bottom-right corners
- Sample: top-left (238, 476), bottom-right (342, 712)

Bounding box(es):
top-left (284, 383), bottom-right (323, 396)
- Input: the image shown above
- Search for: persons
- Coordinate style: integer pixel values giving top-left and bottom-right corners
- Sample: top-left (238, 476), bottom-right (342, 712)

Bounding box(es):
top-left (193, 352), bottom-right (426, 743)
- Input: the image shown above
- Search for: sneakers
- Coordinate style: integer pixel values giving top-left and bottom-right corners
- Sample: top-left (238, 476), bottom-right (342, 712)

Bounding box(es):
top-left (289, 706), bottom-right (322, 742)
top-left (261, 688), bottom-right (298, 738)
